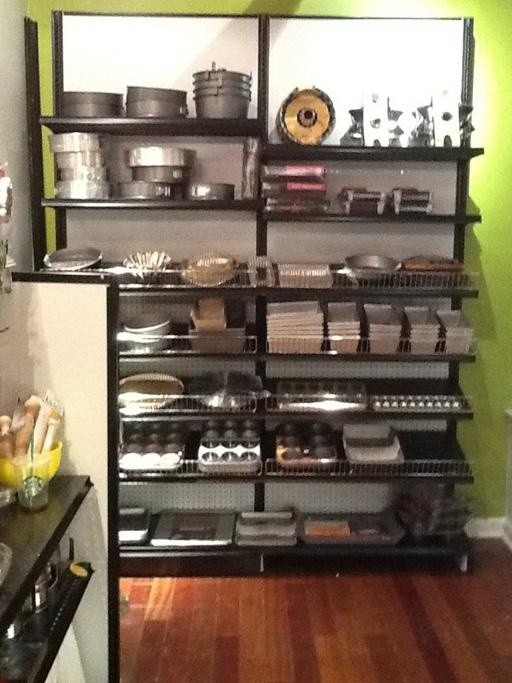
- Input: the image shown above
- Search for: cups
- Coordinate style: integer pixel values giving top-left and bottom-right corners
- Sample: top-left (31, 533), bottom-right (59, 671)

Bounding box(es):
top-left (11, 451), bottom-right (52, 513)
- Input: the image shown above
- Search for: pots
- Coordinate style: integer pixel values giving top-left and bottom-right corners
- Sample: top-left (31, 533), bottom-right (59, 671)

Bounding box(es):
top-left (192, 67), bottom-right (252, 119)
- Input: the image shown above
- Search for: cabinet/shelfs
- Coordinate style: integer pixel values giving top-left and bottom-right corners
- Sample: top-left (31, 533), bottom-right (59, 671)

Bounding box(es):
top-left (0, 118), bottom-right (482, 683)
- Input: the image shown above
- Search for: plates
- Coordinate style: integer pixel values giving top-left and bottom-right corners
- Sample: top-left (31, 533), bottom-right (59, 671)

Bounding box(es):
top-left (118, 372), bottom-right (187, 410)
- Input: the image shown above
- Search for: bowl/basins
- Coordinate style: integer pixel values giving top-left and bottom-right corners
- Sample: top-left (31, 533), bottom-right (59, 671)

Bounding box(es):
top-left (121, 249), bottom-right (172, 279)
top-left (343, 251), bottom-right (401, 278)
top-left (1, 437), bottom-right (63, 491)
